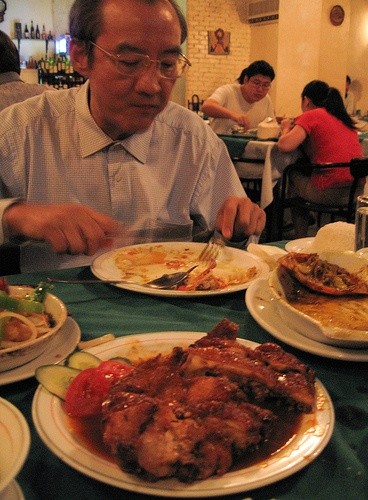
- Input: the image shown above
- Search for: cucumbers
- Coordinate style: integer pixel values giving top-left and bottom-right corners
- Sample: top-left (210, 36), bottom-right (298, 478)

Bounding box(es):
top-left (108, 356), bottom-right (131, 364)
top-left (35, 363), bottom-right (82, 400)
top-left (66, 351), bottom-right (102, 370)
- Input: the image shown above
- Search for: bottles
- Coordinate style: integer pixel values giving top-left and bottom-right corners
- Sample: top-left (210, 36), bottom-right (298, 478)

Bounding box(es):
top-left (26, 54), bottom-right (87, 91)
top-left (25, 20), bottom-right (54, 39)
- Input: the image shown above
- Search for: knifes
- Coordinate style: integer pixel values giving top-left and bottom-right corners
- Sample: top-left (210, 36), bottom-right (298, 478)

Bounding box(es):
top-left (103, 226), bottom-right (206, 238)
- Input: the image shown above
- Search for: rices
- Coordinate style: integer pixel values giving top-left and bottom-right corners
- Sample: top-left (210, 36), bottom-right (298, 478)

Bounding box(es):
top-left (301, 221), bottom-right (355, 252)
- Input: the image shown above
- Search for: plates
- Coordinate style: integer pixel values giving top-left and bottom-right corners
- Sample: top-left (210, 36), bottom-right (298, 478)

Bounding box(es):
top-left (0, 283), bottom-right (67, 373)
top-left (91, 242), bottom-right (270, 297)
top-left (0, 480), bottom-right (25, 500)
top-left (284, 237), bottom-right (315, 253)
top-left (0, 315), bottom-right (81, 386)
top-left (32, 331), bottom-right (335, 498)
top-left (269, 249), bottom-right (368, 352)
top-left (245, 273), bottom-right (368, 362)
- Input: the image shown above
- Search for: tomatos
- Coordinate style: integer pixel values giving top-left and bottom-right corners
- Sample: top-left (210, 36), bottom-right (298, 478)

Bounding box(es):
top-left (65, 361), bottom-right (134, 417)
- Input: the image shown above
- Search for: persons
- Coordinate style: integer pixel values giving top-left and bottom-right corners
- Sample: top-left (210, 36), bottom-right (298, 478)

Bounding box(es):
top-left (277, 80), bottom-right (367, 238)
top-left (0, 0), bottom-right (266, 275)
top-left (200, 60), bottom-right (276, 135)
top-left (0, 30), bottom-right (57, 112)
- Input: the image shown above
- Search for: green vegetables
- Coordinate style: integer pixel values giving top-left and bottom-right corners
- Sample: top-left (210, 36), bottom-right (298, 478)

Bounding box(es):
top-left (0, 281), bottom-right (57, 340)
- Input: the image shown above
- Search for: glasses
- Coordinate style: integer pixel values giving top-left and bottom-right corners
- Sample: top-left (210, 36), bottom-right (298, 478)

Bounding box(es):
top-left (250, 79), bottom-right (271, 90)
top-left (87, 39), bottom-right (192, 80)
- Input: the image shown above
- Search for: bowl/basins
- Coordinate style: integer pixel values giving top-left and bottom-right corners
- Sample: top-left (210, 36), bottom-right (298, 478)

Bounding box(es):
top-left (0, 397), bottom-right (30, 494)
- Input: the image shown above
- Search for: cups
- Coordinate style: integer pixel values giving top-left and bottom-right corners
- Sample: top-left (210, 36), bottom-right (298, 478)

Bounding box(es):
top-left (284, 114), bottom-right (294, 127)
top-left (354, 195), bottom-right (368, 252)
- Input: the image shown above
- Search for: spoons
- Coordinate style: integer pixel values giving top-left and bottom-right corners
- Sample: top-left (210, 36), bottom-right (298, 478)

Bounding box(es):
top-left (46, 272), bottom-right (189, 289)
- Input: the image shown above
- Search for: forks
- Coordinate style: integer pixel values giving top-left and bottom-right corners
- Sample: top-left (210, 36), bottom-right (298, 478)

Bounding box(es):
top-left (198, 231), bottom-right (225, 265)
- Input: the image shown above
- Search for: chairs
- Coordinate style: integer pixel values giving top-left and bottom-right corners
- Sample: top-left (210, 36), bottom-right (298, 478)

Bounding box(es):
top-left (276, 158), bottom-right (368, 241)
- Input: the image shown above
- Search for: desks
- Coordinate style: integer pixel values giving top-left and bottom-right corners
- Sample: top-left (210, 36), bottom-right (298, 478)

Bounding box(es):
top-left (0, 243), bottom-right (368, 500)
top-left (219, 135), bottom-right (368, 242)
